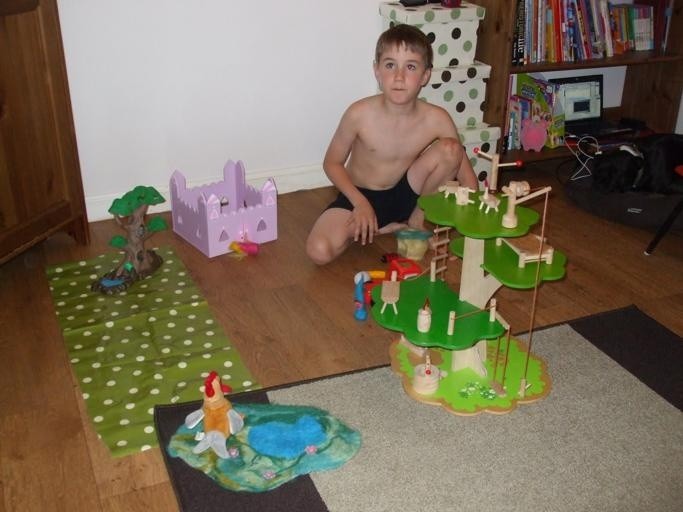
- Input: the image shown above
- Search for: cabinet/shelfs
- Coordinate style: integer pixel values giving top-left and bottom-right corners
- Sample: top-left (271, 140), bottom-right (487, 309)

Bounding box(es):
top-left (473, 0), bottom-right (683, 171)
top-left (0, 1), bottom-right (91, 265)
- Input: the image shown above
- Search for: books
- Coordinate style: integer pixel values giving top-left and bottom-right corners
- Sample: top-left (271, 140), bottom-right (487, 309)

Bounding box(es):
top-left (511, 1), bottom-right (655, 68)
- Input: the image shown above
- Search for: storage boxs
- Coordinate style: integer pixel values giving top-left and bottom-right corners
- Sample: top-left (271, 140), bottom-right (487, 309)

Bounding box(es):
top-left (378, 4), bottom-right (486, 69)
top-left (457, 127), bottom-right (501, 189)
top-left (420, 60), bottom-right (490, 127)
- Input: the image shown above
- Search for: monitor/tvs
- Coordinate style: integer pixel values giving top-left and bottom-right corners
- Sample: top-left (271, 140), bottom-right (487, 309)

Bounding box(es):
top-left (548, 73), bottom-right (603, 137)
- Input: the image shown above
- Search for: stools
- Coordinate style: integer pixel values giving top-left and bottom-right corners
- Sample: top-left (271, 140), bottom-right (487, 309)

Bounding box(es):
top-left (644, 166), bottom-right (683, 256)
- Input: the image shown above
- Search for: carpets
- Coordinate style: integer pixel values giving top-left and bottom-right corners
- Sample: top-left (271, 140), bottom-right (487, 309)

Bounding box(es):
top-left (151, 302), bottom-right (683, 512)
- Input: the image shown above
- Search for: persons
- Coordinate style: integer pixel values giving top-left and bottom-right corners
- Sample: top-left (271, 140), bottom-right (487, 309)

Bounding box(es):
top-left (304, 25), bottom-right (479, 266)
